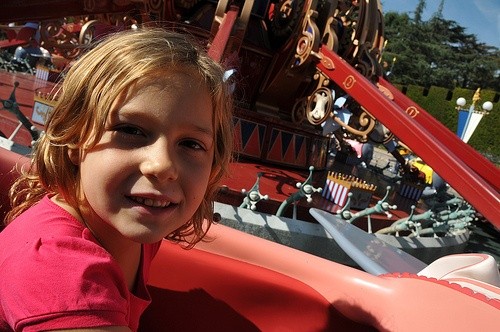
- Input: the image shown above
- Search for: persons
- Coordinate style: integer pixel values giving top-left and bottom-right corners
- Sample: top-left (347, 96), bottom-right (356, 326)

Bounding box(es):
top-left (0, 29), bottom-right (239, 332)
